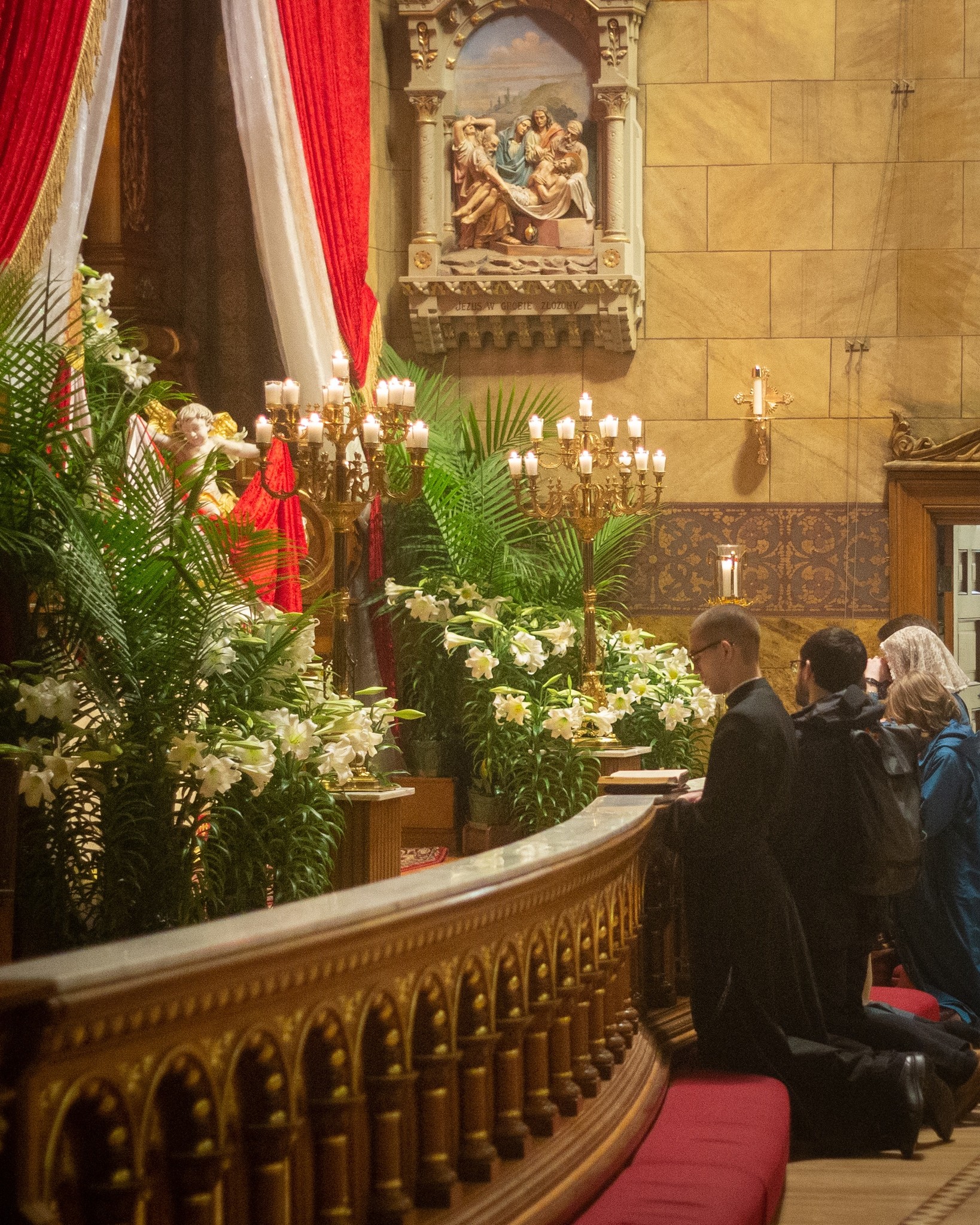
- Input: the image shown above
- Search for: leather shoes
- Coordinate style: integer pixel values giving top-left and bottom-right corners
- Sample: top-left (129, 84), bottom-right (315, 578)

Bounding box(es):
top-left (923, 1057), bottom-right (955, 1142)
top-left (892, 1052), bottom-right (926, 1158)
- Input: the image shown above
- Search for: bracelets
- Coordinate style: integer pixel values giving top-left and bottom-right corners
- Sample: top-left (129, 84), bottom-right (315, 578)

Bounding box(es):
top-left (866, 677), bottom-right (880, 688)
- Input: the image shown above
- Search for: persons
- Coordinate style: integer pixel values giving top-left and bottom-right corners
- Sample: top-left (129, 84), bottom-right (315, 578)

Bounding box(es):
top-left (667, 603), bottom-right (956, 1161)
top-left (862, 613), bottom-right (980, 1049)
top-left (774, 625), bottom-right (980, 1119)
top-left (451, 105), bottom-right (596, 251)
top-left (140, 403), bottom-right (285, 516)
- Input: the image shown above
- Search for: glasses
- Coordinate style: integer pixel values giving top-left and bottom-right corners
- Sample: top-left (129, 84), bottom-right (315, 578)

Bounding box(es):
top-left (790, 660), bottom-right (804, 673)
top-left (688, 640), bottom-right (721, 664)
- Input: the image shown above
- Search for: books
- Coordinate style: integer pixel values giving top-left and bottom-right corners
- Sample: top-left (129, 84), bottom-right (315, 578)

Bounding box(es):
top-left (596, 768), bottom-right (689, 787)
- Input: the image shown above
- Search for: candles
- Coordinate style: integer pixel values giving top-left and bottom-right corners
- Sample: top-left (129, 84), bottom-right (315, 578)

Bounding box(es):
top-left (413, 421), bottom-right (428, 447)
top-left (308, 412), bottom-right (323, 443)
top-left (328, 378), bottom-right (344, 404)
top-left (652, 449), bottom-right (665, 472)
top-left (524, 452), bottom-right (538, 475)
top-left (508, 452), bottom-right (522, 474)
top-left (264, 381), bottom-right (282, 405)
top-left (579, 393), bottom-right (593, 417)
top-left (283, 379), bottom-right (300, 405)
top-left (557, 420), bottom-right (561, 438)
top-left (618, 451), bottom-right (633, 473)
top-left (298, 418), bottom-right (308, 446)
top-left (333, 350), bottom-right (348, 379)
top-left (562, 417), bottom-right (575, 438)
top-left (721, 554), bottom-right (739, 597)
top-left (751, 365), bottom-right (764, 414)
top-left (375, 381), bottom-right (389, 407)
top-left (363, 415), bottom-right (384, 450)
top-left (388, 377), bottom-right (404, 405)
top-left (406, 424), bottom-right (412, 446)
top-left (598, 418), bottom-right (607, 437)
top-left (606, 414), bottom-right (618, 437)
top-left (579, 450), bottom-right (592, 473)
top-left (402, 380), bottom-right (417, 406)
top-left (634, 448), bottom-right (649, 470)
top-left (529, 415), bottom-right (543, 438)
top-left (255, 415), bottom-right (273, 443)
top-left (626, 415), bottom-right (643, 437)
top-left (323, 385), bottom-right (328, 405)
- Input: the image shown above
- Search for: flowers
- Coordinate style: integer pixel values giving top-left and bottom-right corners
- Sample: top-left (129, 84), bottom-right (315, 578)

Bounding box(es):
top-left (0, 253), bottom-right (720, 964)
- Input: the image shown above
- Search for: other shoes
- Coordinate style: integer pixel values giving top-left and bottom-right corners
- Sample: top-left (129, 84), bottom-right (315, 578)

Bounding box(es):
top-left (951, 1048), bottom-right (980, 1125)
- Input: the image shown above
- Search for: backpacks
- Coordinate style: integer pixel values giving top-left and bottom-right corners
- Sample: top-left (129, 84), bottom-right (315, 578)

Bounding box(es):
top-left (846, 722), bottom-right (922, 896)
